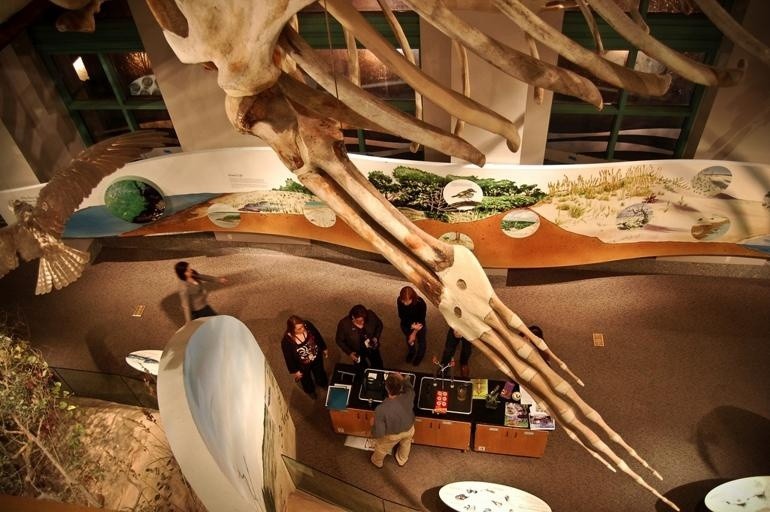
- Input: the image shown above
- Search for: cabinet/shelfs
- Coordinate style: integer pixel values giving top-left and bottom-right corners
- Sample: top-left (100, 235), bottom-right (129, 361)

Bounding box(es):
top-left (329, 407), bottom-right (548, 457)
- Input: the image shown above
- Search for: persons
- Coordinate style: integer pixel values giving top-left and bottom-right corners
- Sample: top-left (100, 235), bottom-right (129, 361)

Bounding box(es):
top-left (519, 326), bottom-right (550, 367)
top-left (440, 327), bottom-right (472, 378)
top-left (370, 373), bottom-right (416, 469)
top-left (281, 315), bottom-right (328, 401)
top-left (175, 262), bottom-right (228, 323)
top-left (397, 286), bottom-right (427, 366)
top-left (335, 304), bottom-right (383, 370)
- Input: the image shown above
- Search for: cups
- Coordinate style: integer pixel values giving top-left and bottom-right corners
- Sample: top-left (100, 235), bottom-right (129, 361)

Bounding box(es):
top-left (457, 385), bottom-right (467, 401)
